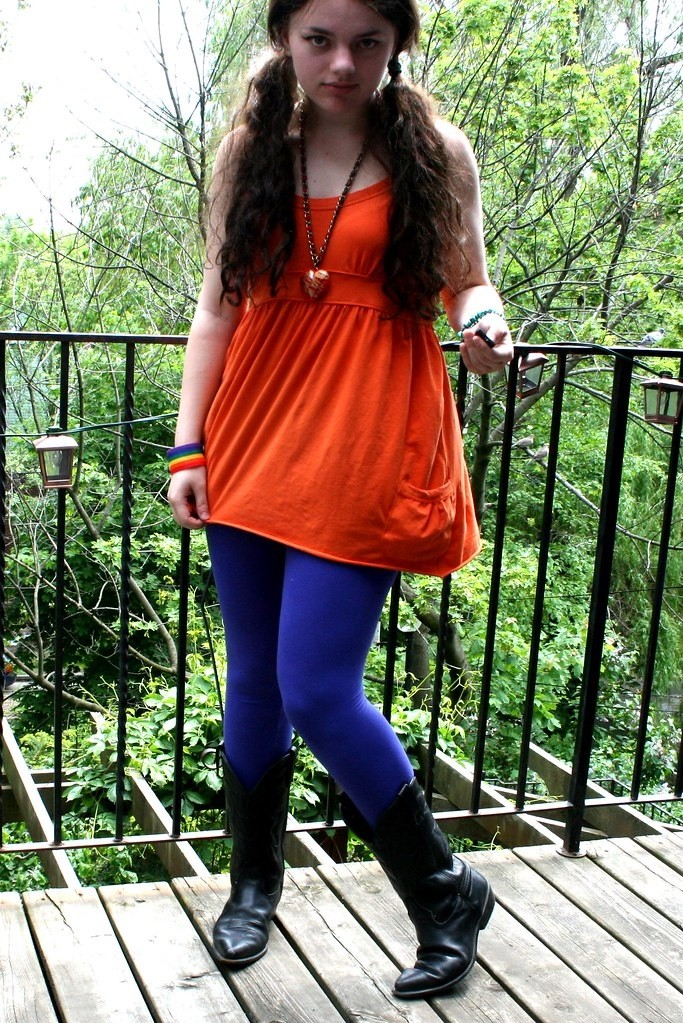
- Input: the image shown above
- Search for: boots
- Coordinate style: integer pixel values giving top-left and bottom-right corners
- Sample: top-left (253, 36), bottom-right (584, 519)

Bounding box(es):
top-left (210, 738), bottom-right (298, 961)
top-left (338, 776), bottom-right (493, 996)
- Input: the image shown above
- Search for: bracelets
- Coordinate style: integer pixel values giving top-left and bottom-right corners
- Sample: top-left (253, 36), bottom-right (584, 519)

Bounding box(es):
top-left (457, 309), bottom-right (509, 342)
top-left (166, 442), bottom-right (207, 476)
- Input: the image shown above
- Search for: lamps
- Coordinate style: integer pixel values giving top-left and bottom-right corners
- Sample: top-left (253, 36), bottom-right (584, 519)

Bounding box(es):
top-left (639, 370), bottom-right (683, 425)
top-left (31, 426), bottom-right (80, 489)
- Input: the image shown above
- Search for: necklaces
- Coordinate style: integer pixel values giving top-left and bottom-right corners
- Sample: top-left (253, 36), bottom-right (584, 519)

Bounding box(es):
top-left (300, 88), bottom-right (382, 301)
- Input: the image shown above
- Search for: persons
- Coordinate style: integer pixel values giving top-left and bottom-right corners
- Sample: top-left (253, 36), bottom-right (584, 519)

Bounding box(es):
top-left (167, 0), bottom-right (514, 1001)
